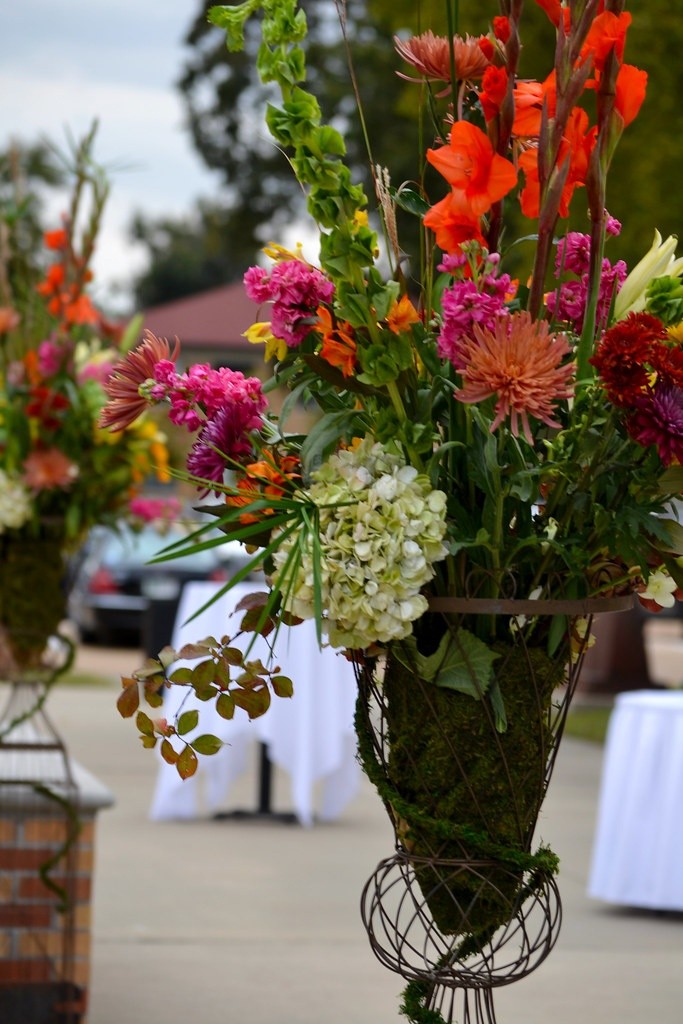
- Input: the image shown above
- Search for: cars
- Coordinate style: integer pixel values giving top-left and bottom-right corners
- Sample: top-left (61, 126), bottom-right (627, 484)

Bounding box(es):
top-left (65, 522), bottom-right (235, 649)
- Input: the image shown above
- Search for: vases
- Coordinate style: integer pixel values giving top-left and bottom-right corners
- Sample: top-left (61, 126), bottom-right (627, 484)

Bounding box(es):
top-left (0, 526), bottom-right (90, 1024)
top-left (338, 556), bottom-right (636, 1023)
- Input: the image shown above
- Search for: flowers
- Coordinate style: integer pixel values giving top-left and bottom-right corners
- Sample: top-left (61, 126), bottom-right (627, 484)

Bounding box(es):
top-left (0, 123), bottom-right (187, 685)
top-left (97, 0), bottom-right (682, 781)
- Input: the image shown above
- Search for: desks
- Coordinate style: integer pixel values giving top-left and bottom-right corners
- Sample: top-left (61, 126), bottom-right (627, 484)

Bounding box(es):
top-left (158, 581), bottom-right (360, 827)
top-left (589, 692), bottom-right (683, 914)
